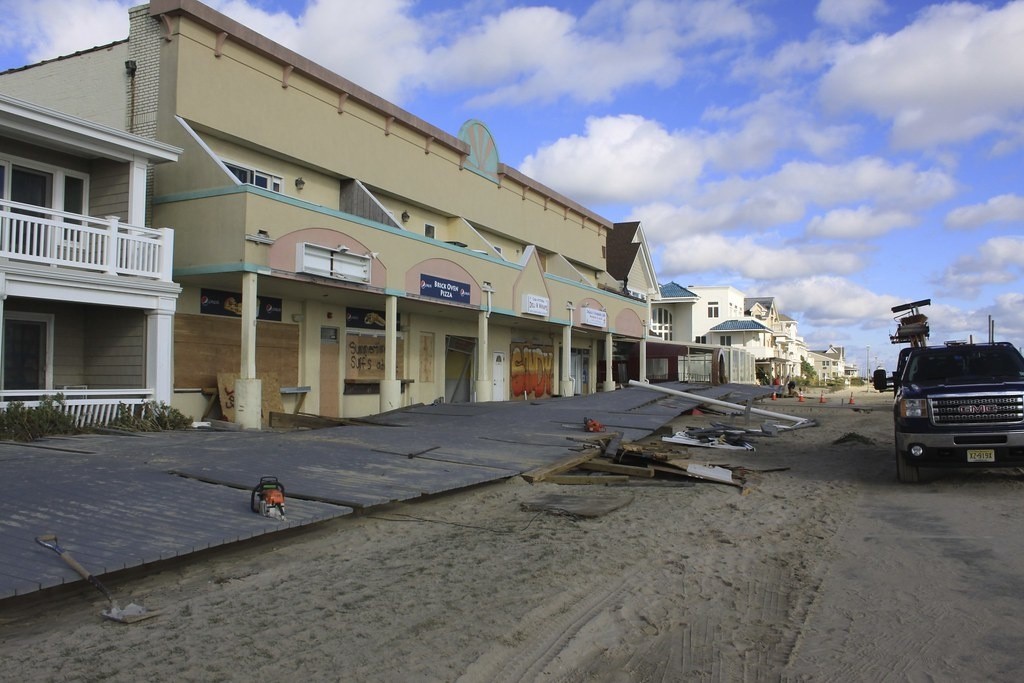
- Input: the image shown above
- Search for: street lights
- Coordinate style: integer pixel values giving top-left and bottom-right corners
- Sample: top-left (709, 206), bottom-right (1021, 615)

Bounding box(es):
top-left (866, 345), bottom-right (870, 392)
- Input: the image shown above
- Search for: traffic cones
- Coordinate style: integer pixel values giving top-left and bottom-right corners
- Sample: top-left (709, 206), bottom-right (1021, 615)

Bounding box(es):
top-left (798, 390), bottom-right (805, 402)
top-left (819, 390), bottom-right (826, 403)
top-left (772, 391), bottom-right (776, 400)
top-left (848, 392), bottom-right (855, 404)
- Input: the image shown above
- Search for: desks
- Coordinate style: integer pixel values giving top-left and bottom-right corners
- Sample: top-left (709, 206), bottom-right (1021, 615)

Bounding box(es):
top-left (54, 385), bottom-right (87, 399)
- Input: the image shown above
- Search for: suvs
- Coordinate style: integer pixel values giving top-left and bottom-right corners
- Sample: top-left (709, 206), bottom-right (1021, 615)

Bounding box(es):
top-left (892, 340), bottom-right (1024, 484)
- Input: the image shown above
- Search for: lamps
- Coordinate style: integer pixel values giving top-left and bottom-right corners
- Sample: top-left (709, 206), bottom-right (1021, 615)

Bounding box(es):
top-left (402, 210), bottom-right (409, 222)
top-left (292, 314), bottom-right (305, 322)
top-left (335, 245), bottom-right (379, 260)
top-left (295, 177), bottom-right (305, 189)
top-left (125, 60), bottom-right (137, 75)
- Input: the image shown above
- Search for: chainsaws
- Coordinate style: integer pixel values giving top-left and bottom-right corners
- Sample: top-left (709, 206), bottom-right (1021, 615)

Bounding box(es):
top-left (251, 476), bottom-right (286, 523)
top-left (561, 417), bottom-right (607, 433)
